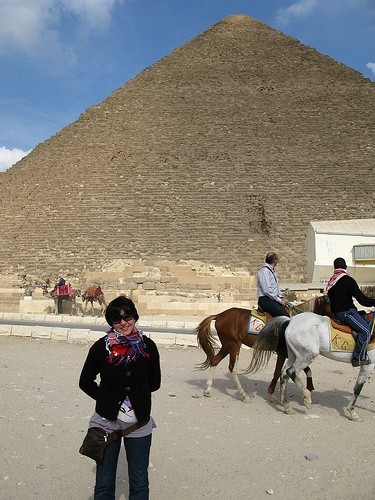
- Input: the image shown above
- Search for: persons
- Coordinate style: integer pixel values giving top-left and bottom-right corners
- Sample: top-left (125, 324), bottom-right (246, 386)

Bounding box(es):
top-left (80, 297), bottom-right (161, 500)
top-left (327, 257), bottom-right (375, 366)
top-left (54, 275), bottom-right (65, 289)
top-left (256, 252), bottom-right (291, 317)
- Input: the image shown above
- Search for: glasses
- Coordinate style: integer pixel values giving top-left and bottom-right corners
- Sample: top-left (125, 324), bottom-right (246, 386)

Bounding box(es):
top-left (111, 313), bottom-right (133, 324)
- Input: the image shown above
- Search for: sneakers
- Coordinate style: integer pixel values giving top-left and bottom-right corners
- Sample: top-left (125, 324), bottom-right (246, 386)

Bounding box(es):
top-left (352, 359), bottom-right (371, 367)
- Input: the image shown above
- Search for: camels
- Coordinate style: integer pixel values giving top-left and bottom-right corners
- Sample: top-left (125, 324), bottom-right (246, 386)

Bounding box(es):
top-left (81, 286), bottom-right (108, 317)
top-left (42, 283), bottom-right (81, 315)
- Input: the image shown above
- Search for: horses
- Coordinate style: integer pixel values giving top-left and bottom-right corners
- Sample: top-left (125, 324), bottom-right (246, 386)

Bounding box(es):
top-left (233, 312), bottom-right (375, 422)
top-left (193, 295), bottom-right (330, 402)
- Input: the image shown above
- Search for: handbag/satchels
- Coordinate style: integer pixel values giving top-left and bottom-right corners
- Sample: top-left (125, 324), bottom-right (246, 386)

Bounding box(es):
top-left (79, 427), bottom-right (116, 461)
